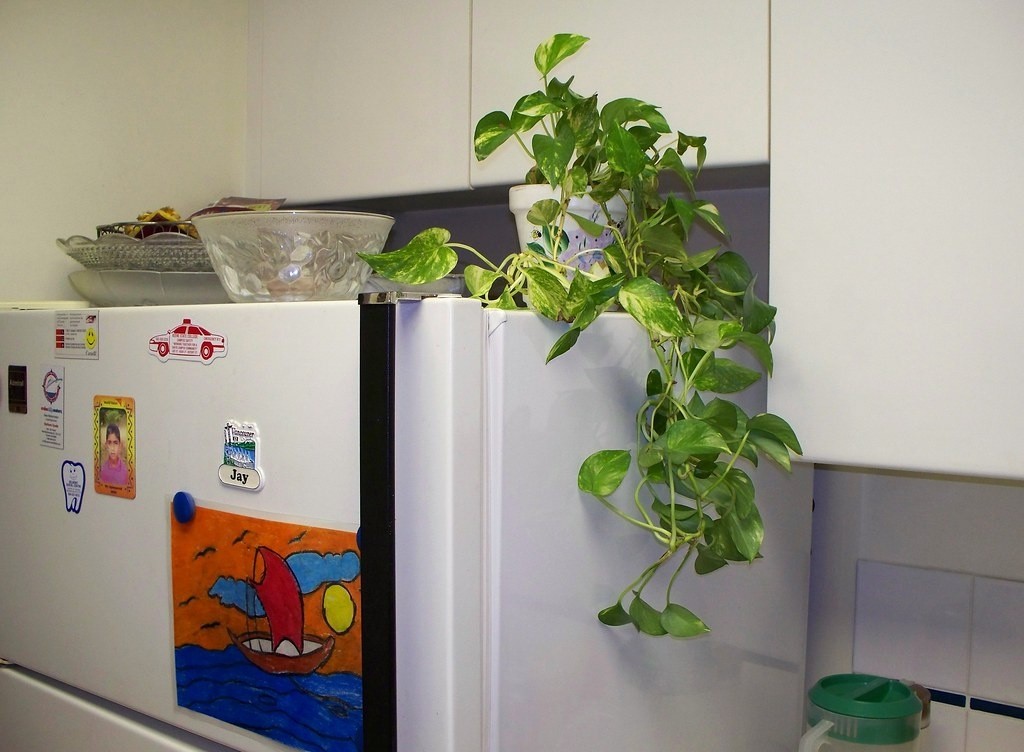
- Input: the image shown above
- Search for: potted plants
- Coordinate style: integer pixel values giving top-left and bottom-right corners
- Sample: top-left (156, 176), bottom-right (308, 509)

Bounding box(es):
top-left (355, 30), bottom-right (803, 635)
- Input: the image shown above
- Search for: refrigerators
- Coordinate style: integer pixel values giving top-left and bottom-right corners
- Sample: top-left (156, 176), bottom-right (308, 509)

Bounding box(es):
top-left (1, 303), bottom-right (815, 752)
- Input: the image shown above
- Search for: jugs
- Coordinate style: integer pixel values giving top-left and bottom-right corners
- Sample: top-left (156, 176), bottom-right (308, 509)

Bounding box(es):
top-left (797, 674), bottom-right (922, 752)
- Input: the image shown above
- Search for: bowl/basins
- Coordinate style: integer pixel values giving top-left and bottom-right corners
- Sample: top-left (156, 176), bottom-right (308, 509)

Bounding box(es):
top-left (191, 209), bottom-right (396, 303)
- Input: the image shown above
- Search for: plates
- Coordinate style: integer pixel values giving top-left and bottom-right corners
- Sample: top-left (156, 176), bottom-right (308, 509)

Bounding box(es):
top-left (68, 270), bottom-right (228, 306)
top-left (54, 234), bottom-right (214, 272)
